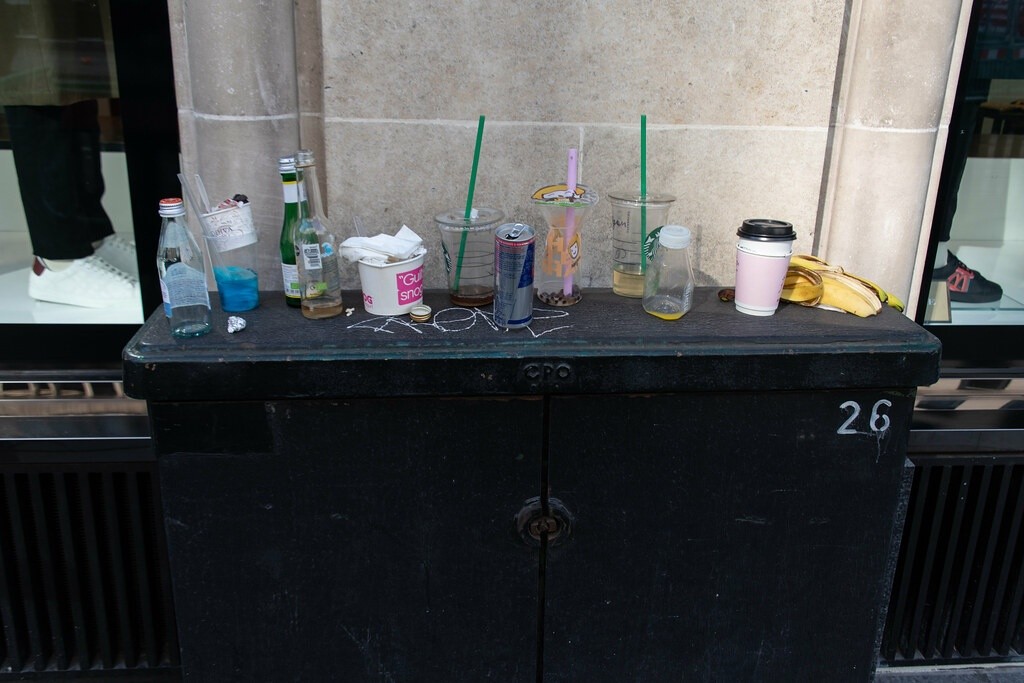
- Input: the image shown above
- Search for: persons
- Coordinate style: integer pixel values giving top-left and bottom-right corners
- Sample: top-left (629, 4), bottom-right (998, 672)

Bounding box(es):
top-left (930, 0), bottom-right (1003, 304)
top-left (0, 0), bottom-right (143, 312)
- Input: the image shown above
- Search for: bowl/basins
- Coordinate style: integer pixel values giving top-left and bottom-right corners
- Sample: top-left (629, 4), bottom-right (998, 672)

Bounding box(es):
top-left (358, 249), bottom-right (428, 316)
top-left (203, 203), bottom-right (258, 253)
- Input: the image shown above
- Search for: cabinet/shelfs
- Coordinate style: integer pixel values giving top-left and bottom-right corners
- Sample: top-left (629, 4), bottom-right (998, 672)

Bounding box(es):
top-left (124, 289), bottom-right (941, 683)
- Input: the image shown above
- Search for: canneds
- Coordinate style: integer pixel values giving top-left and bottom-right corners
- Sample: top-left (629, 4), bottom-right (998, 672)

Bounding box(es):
top-left (493, 222), bottom-right (537, 329)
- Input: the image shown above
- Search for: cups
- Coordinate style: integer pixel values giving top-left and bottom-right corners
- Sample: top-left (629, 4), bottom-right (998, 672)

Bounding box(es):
top-left (434, 207), bottom-right (506, 307)
top-left (735, 219), bottom-right (797, 317)
top-left (532, 183), bottom-right (599, 307)
top-left (204, 230), bottom-right (259, 313)
top-left (607, 190), bottom-right (676, 299)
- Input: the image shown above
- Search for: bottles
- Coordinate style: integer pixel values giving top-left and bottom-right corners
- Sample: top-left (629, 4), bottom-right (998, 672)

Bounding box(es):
top-left (293, 150), bottom-right (343, 319)
top-left (157, 199), bottom-right (213, 338)
top-left (279, 155), bottom-right (303, 309)
top-left (643, 225), bottom-right (694, 320)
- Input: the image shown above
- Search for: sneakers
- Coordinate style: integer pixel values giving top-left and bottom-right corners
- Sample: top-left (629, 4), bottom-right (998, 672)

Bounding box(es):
top-left (932, 248), bottom-right (1004, 302)
top-left (90, 233), bottom-right (139, 276)
top-left (29, 256), bottom-right (140, 310)
